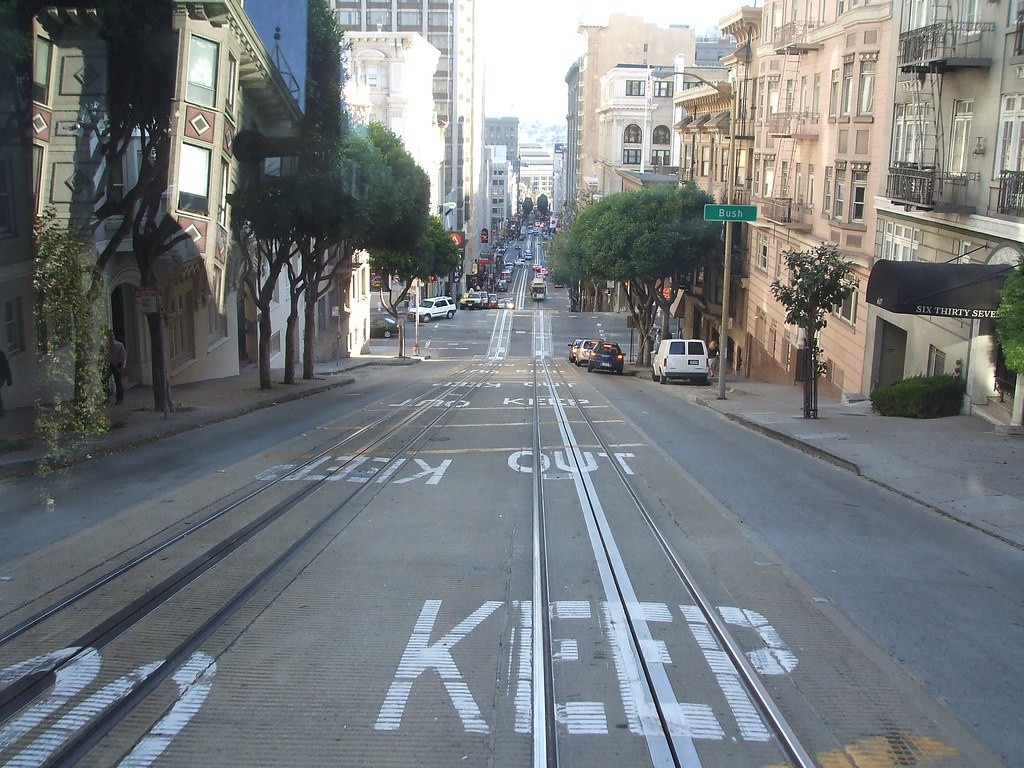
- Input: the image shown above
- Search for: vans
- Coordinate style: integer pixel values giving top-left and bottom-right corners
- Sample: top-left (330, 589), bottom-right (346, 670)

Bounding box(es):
top-left (650, 338), bottom-right (709, 386)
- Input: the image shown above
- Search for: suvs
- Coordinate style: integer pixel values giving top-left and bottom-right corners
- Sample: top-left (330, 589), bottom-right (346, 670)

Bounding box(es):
top-left (406, 296), bottom-right (456, 323)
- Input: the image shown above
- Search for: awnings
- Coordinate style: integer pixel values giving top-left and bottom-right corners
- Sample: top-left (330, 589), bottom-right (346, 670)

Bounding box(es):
top-left (865, 257), bottom-right (1018, 319)
top-left (669, 288), bottom-right (686, 318)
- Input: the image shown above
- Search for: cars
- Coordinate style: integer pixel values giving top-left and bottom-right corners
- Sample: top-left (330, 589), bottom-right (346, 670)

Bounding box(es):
top-left (518, 220), bottom-right (547, 260)
top-left (459, 291), bottom-right (516, 310)
top-left (370, 314), bottom-right (399, 338)
top-left (514, 259), bottom-right (564, 288)
top-left (567, 338), bottom-right (627, 375)
top-left (496, 263), bottom-right (514, 292)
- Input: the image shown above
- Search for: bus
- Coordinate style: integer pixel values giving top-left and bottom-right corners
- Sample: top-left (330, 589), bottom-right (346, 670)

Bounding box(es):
top-left (530, 278), bottom-right (546, 301)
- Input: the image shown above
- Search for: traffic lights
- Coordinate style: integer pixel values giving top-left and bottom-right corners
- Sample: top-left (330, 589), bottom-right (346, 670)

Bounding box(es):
top-left (480, 228), bottom-right (488, 244)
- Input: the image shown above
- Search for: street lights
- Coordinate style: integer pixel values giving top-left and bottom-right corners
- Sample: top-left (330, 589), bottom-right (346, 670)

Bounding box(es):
top-left (651, 70), bottom-right (737, 402)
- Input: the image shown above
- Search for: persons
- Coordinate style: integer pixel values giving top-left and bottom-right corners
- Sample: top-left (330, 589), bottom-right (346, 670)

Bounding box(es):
top-left (649, 324), bottom-right (672, 354)
top-left (707, 341), bottom-right (717, 377)
